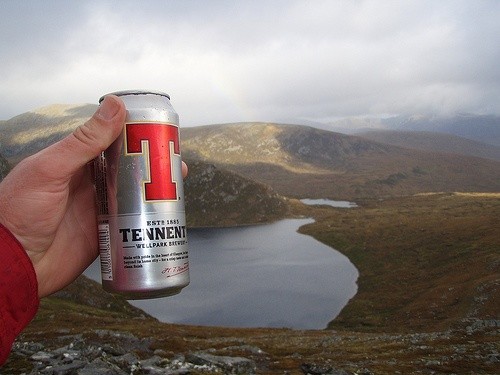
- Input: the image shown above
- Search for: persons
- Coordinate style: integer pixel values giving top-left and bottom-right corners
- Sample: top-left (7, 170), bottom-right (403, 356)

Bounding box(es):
top-left (0, 96), bottom-right (189, 369)
